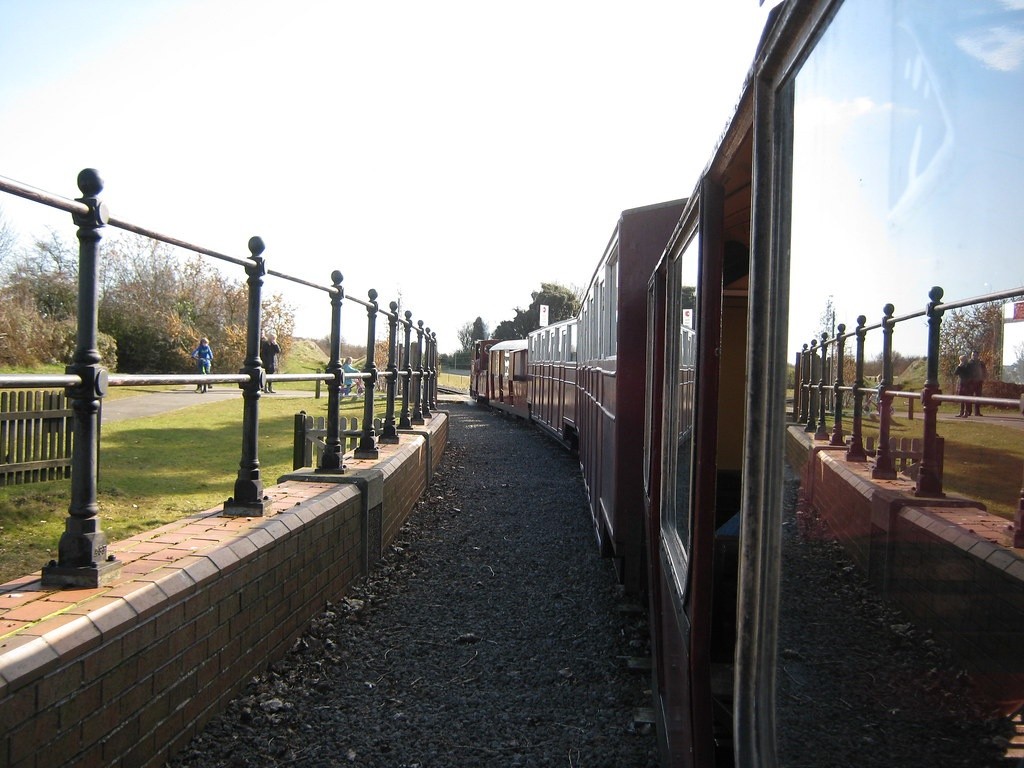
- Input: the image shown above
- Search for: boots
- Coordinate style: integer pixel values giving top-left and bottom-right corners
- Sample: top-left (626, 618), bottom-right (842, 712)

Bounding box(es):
top-left (264, 387), bottom-right (270, 393)
top-left (268, 386), bottom-right (276, 393)
top-left (955, 410), bottom-right (964, 417)
top-left (962, 410), bottom-right (968, 418)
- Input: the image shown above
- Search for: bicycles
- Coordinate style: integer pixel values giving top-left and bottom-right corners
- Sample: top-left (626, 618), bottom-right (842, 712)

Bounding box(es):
top-left (338, 378), bottom-right (365, 401)
top-left (862, 392), bottom-right (894, 416)
top-left (192, 357), bottom-right (212, 393)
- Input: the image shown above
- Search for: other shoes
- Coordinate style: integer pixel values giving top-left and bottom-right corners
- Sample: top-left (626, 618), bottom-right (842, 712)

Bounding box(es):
top-left (196, 388), bottom-right (199, 390)
top-left (968, 412), bottom-right (971, 416)
top-left (208, 386), bottom-right (212, 388)
top-left (975, 412), bottom-right (983, 416)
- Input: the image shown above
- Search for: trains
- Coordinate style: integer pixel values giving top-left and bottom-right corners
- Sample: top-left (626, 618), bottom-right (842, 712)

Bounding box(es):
top-left (469, 0), bottom-right (1024, 768)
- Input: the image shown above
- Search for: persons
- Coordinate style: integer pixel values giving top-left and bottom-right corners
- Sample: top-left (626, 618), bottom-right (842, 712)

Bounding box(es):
top-left (956, 350), bottom-right (987, 418)
top-left (341, 357), bottom-right (361, 397)
top-left (191, 338), bottom-right (213, 393)
top-left (261, 328), bottom-right (282, 393)
top-left (869, 375), bottom-right (893, 411)
